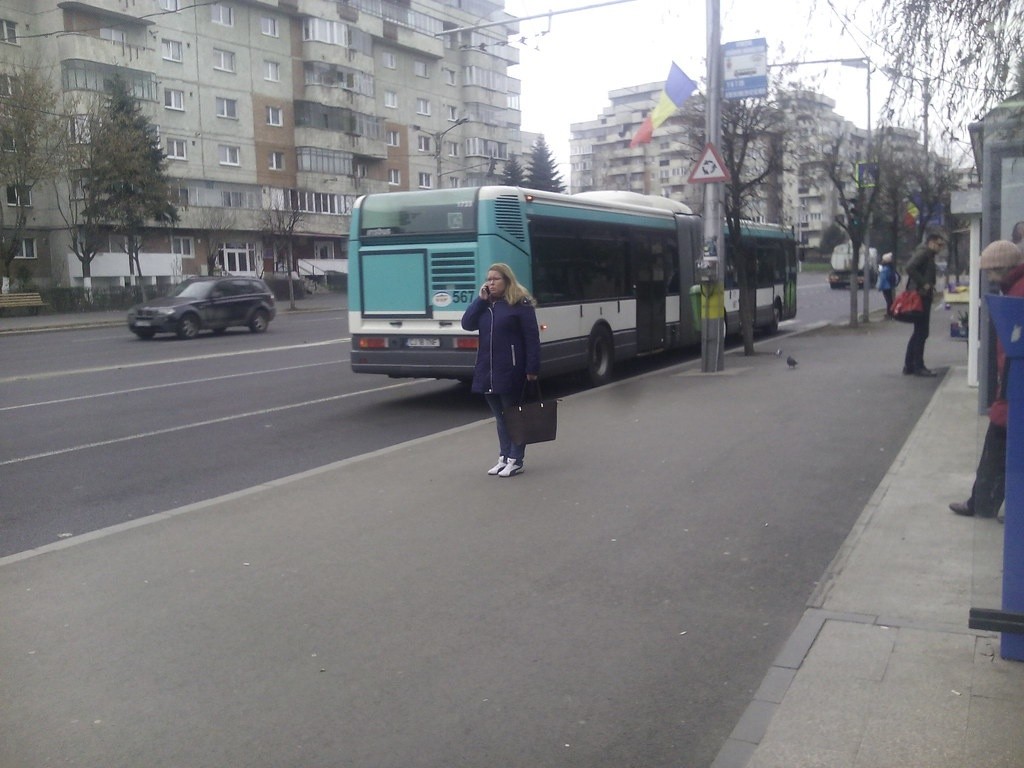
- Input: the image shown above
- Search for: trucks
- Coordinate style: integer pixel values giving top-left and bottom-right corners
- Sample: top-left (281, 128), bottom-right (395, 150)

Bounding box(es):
top-left (828, 244), bottom-right (879, 289)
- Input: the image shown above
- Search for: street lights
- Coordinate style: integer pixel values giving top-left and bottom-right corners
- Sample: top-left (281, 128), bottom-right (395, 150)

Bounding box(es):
top-left (406, 115), bottom-right (469, 187)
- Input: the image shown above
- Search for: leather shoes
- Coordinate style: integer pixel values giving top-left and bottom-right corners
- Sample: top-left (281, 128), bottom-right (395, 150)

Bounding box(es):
top-left (901, 365), bottom-right (939, 378)
top-left (947, 501), bottom-right (994, 518)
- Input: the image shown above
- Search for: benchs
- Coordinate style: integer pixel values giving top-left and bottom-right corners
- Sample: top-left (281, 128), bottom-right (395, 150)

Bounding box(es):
top-left (0, 292), bottom-right (46, 317)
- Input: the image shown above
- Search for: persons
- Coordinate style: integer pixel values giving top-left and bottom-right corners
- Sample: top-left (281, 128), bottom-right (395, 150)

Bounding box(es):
top-left (875, 251), bottom-right (895, 322)
top-left (462, 263), bottom-right (541, 476)
top-left (1011, 221), bottom-right (1024, 252)
top-left (947, 239), bottom-right (1024, 521)
top-left (902, 234), bottom-right (944, 376)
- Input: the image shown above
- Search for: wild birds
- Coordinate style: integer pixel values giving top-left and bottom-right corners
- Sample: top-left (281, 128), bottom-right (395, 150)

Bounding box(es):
top-left (775, 348), bottom-right (782, 359)
top-left (787, 355), bottom-right (799, 369)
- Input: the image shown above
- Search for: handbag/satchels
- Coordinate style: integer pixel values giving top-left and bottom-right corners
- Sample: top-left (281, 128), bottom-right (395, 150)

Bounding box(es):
top-left (889, 289), bottom-right (922, 323)
top-left (504, 377), bottom-right (558, 445)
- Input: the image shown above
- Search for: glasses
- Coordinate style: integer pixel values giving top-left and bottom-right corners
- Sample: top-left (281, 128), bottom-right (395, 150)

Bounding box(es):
top-left (486, 278), bottom-right (502, 282)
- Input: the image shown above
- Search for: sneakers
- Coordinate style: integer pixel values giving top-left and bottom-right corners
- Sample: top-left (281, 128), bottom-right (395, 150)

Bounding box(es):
top-left (488, 455), bottom-right (506, 474)
top-left (498, 457), bottom-right (524, 476)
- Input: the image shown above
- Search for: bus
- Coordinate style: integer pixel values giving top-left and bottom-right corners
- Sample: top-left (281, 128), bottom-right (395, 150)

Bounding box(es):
top-left (347, 186), bottom-right (799, 388)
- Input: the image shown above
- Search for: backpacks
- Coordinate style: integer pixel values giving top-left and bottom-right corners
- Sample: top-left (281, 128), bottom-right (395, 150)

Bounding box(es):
top-left (891, 268), bottom-right (901, 287)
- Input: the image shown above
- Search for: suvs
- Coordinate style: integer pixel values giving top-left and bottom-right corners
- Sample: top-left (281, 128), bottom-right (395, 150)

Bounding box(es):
top-left (126, 277), bottom-right (278, 342)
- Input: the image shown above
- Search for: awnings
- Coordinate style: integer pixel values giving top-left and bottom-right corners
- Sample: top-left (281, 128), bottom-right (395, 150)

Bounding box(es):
top-left (298, 259), bottom-right (348, 276)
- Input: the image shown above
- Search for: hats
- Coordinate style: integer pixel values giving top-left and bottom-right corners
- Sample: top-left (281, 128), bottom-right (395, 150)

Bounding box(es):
top-left (980, 240), bottom-right (1024, 269)
top-left (882, 252), bottom-right (894, 262)
